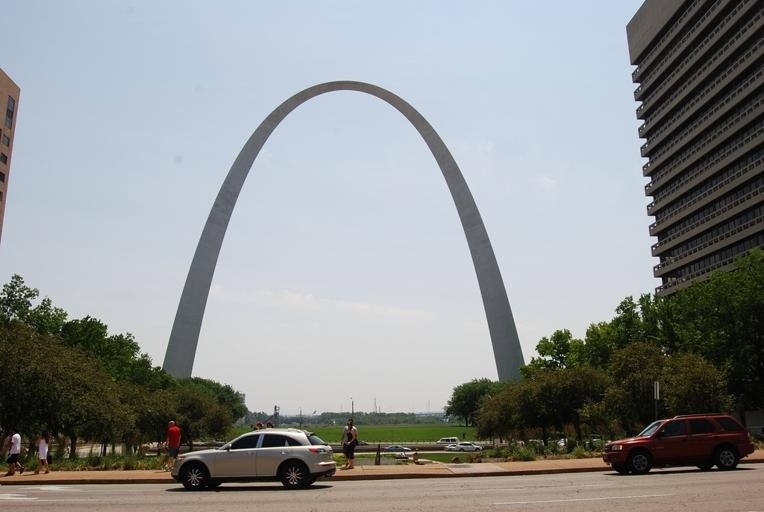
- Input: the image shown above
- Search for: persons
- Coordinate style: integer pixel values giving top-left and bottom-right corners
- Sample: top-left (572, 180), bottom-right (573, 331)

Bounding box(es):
top-left (34, 430), bottom-right (49, 474)
top-left (345, 421), bottom-right (356, 470)
top-left (254, 423), bottom-right (273, 430)
top-left (341, 419), bottom-right (358, 466)
top-left (5, 428), bottom-right (24, 476)
top-left (166, 421), bottom-right (181, 471)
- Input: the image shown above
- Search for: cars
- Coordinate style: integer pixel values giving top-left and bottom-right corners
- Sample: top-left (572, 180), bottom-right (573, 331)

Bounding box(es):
top-left (445, 444), bottom-right (457, 451)
top-left (384, 445), bottom-right (412, 453)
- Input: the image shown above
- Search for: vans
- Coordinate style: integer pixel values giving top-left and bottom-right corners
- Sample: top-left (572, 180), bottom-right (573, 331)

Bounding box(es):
top-left (437, 437), bottom-right (459, 445)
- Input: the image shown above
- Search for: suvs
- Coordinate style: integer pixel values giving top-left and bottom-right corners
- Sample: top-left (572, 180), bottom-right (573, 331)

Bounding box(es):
top-left (456, 442), bottom-right (482, 452)
top-left (170, 426), bottom-right (337, 490)
top-left (601, 410), bottom-right (755, 476)
